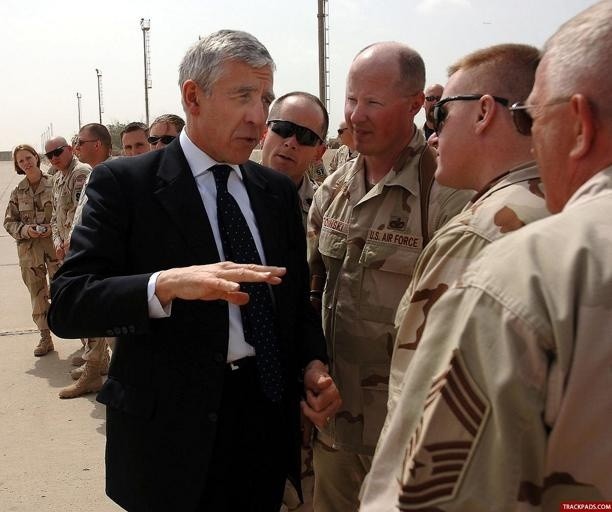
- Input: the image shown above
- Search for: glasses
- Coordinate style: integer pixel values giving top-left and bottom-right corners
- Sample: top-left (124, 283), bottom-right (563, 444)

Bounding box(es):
top-left (147, 134), bottom-right (175, 145)
top-left (507, 97), bottom-right (573, 135)
top-left (45, 145), bottom-right (69, 159)
top-left (267, 119), bottom-right (324, 148)
top-left (425, 95), bottom-right (441, 101)
top-left (433, 95), bottom-right (509, 137)
top-left (73, 136), bottom-right (98, 146)
top-left (338, 127), bottom-right (348, 136)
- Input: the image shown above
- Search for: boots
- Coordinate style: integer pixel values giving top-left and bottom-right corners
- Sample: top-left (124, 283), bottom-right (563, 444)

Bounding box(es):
top-left (59, 363), bottom-right (104, 399)
top-left (71, 343), bottom-right (110, 380)
top-left (34, 329), bottom-right (54, 356)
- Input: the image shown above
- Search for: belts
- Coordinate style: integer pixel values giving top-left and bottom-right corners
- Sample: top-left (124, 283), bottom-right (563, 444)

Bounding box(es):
top-left (224, 354), bottom-right (257, 374)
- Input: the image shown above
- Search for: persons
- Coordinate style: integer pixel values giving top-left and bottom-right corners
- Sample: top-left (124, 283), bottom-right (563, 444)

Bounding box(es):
top-left (302, 38), bottom-right (479, 512)
top-left (355, 40), bottom-right (556, 512)
top-left (348, 0), bottom-right (612, 512)
top-left (45, 30), bottom-right (343, 511)
top-left (0, 83), bottom-right (475, 400)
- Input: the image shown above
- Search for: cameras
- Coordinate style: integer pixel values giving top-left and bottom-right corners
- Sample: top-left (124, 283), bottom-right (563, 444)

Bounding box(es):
top-left (36, 226), bottom-right (47, 233)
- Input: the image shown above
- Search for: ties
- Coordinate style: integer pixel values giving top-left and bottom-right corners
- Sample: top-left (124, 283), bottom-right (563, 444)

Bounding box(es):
top-left (206, 164), bottom-right (284, 386)
